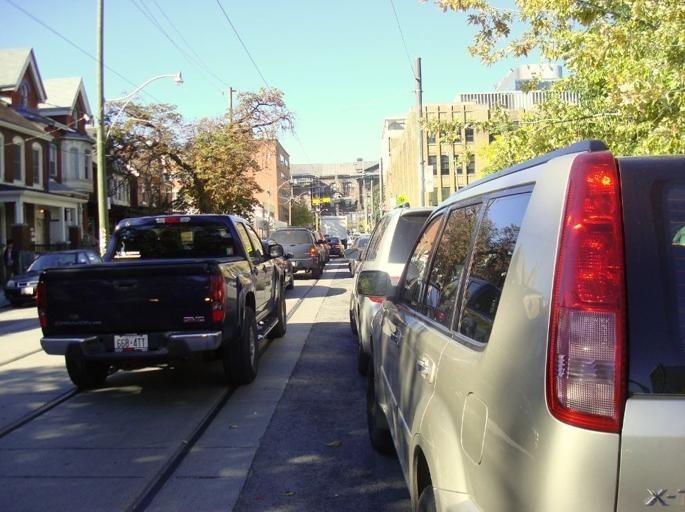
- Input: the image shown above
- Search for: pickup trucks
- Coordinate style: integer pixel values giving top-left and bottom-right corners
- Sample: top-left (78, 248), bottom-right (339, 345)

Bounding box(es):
top-left (36, 213), bottom-right (287, 389)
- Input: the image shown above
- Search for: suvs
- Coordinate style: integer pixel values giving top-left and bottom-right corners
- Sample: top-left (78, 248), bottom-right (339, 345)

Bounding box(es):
top-left (344, 204), bottom-right (434, 376)
top-left (367, 141), bottom-right (684, 512)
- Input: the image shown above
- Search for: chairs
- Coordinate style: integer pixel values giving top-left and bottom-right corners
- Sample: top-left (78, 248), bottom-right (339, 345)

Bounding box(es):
top-left (128, 227), bottom-right (227, 255)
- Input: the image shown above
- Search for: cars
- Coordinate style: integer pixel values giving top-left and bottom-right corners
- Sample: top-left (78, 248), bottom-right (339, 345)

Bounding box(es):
top-left (5, 248), bottom-right (103, 308)
top-left (267, 225), bottom-right (372, 281)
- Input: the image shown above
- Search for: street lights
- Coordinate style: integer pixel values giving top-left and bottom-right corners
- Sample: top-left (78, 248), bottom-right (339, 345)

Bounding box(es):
top-left (276, 177), bottom-right (328, 225)
top-left (98, 71), bottom-right (185, 257)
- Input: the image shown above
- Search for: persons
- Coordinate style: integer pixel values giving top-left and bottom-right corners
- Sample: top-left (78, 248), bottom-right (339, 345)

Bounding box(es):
top-left (0, 239), bottom-right (20, 291)
